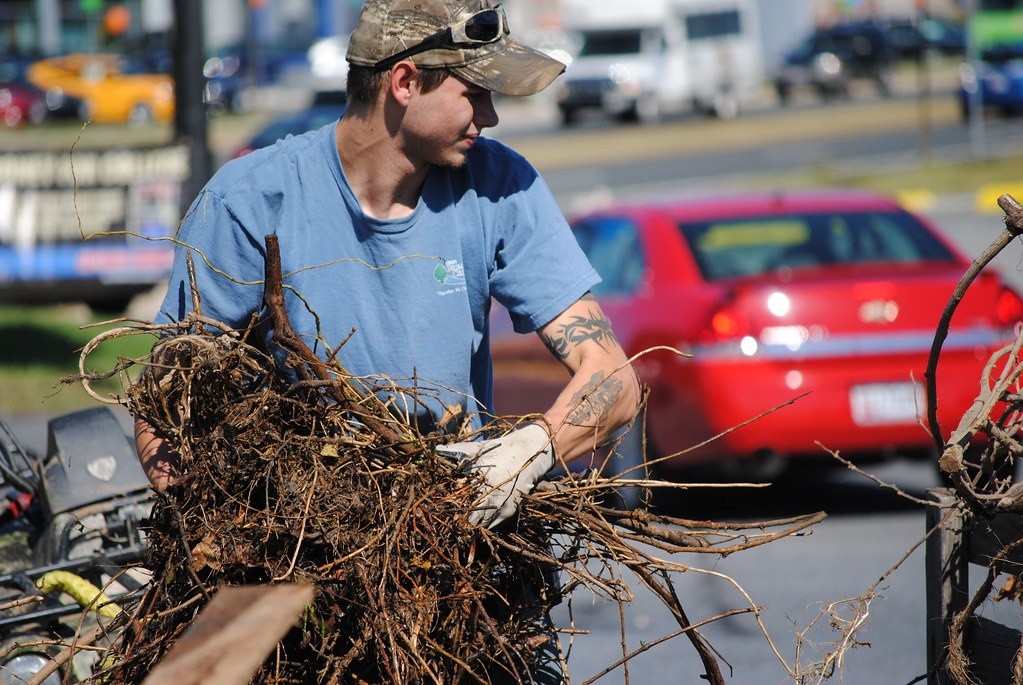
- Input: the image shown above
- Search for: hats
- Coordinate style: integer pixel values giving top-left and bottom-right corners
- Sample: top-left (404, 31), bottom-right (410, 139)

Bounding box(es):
top-left (345, 0), bottom-right (567, 96)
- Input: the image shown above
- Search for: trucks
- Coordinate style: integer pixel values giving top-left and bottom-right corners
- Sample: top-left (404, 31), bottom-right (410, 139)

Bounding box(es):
top-left (553, 0), bottom-right (771, 127)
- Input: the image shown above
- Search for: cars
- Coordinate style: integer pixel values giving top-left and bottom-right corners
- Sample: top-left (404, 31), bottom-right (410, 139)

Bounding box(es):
top-left (483, 188), bottom-right (1023, 512)
top-left (776, 0), bottom-right (1023, 121)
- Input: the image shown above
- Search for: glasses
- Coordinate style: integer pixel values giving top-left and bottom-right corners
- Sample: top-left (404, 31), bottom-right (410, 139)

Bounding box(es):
top-left (374, 3), bottom-right (510, 69)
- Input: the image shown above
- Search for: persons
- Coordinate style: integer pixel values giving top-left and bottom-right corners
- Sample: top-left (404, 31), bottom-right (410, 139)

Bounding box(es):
top-left (135, 0), bottom-right (638, 685)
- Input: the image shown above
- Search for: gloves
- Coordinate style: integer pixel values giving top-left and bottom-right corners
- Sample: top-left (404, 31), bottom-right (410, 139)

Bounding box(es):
top-left (434, 423), bottom-right (556, 531)
